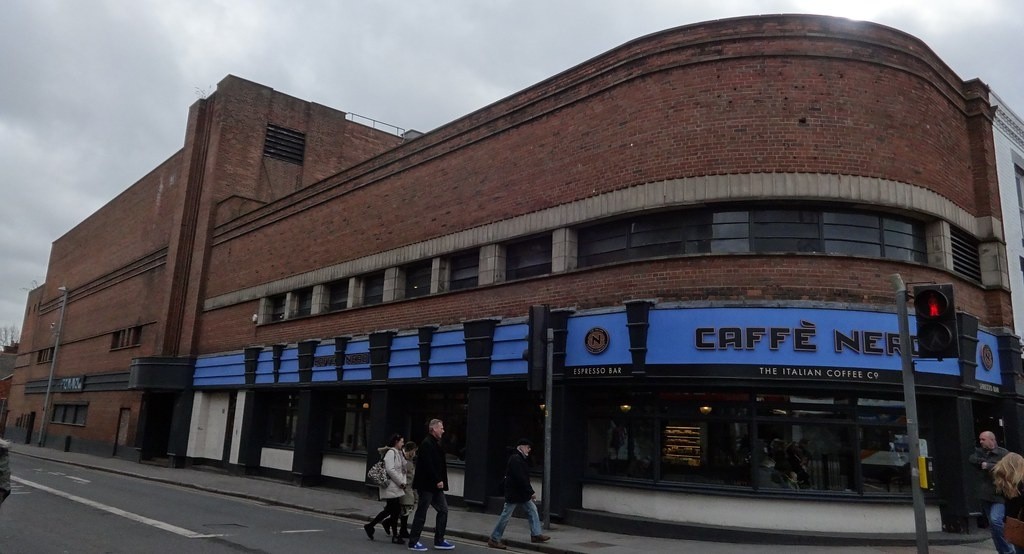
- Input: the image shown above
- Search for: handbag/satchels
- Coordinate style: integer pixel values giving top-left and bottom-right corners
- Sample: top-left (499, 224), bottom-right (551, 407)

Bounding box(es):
top-left (366, 449), bottom-right (396, 488)
top-left (1004, 506), bottom-right (1024, 549)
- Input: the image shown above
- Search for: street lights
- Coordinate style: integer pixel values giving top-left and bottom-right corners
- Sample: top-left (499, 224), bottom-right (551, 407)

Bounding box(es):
top-left (700, 394), bottom-right (718, 482)
top-left (613, 400), bottom-right (639, 476)
top-left (33, 280), bottom-right (70, 449)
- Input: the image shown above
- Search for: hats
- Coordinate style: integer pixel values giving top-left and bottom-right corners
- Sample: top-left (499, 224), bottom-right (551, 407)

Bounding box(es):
top-left (517, 438), bottom-right (534, 446)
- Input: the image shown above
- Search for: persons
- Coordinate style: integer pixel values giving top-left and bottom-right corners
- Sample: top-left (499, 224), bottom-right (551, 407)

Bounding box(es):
top-left (408, 418), bottom-right (455, 551)
top-left (729, 433), bottom-right (814, 490)
top-left (969, 430), bottom-right (1024, 554)
top-left (364, 433), bottom-right (418, 545)
top-left (487, 438), bottom-right (551, 550)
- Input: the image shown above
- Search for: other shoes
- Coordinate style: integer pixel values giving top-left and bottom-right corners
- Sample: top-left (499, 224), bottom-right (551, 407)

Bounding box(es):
top-left (364, 524), bottom-right (375, 539)
top-left (392, 535), bottom-right (405, 544)
top-left (488, 538), bottom-right (506, 549)
top-left (382, 521), bottom-right (390, 534)
top-left (400, 530), bottom-right (410, 537)
top-left (531, 534), bottom-right (551, 542)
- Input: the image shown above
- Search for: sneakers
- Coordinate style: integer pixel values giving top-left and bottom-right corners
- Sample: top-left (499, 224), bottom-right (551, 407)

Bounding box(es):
top-left (434, 539), bottom-right (455, 549)
top-left (408, 542), bottom-right (428, 551)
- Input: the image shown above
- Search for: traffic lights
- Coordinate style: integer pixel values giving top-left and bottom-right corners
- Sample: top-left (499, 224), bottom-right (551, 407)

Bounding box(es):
top-left (915, 286), bottom-right (958, 359)
